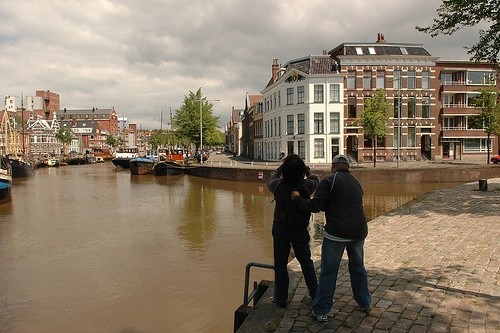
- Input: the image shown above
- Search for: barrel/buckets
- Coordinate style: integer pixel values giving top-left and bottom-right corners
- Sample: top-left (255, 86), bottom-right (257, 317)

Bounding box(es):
top-left (479, 179), bottom-right (487, 191)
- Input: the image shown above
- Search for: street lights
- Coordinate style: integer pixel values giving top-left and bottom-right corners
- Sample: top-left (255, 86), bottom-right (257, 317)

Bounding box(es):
top-left (200, 98), bottom-right (221, 165)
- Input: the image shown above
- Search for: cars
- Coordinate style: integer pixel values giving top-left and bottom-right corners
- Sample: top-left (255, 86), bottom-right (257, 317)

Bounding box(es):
top-left (216, 150), bottom-right (221, 154)
top-left (490, 155), bottom-right (500, 165)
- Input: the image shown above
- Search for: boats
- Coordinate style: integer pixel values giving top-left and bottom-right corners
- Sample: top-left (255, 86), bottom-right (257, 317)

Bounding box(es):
top-left (111, 110), bottom-right (189, 176)
top-left (0, 151), bottom-right (105, 202)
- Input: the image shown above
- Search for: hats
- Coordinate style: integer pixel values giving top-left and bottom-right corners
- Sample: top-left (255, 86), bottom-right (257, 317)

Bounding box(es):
top-left (332, 154), bottom-right (349, 164)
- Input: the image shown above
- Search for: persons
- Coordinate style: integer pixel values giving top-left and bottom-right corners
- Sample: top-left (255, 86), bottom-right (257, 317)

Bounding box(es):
top-left (267, 153), bottom-right (319, 307)
top-left (290, 154), bottom-right (372, 321)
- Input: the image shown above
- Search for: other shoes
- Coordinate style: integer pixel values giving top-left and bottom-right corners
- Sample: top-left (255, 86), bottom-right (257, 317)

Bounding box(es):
top-left (312, 310), bottom-right (328, 322)
top-left (358, 305), bottom-right (369, 312)
